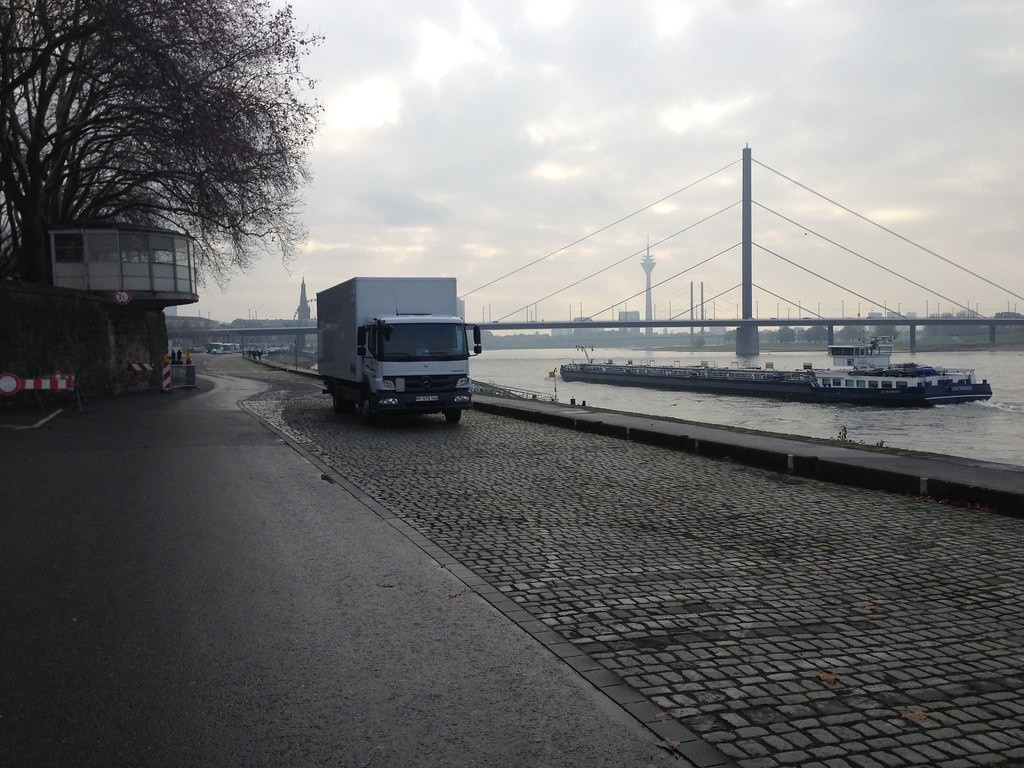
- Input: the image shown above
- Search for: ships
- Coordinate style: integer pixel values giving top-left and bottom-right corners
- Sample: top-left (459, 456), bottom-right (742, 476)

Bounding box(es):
top-left (560, 336), bottom-right (993, 404)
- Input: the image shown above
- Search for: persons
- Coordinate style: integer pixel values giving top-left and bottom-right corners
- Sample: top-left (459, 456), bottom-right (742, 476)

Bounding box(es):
top-left (214, 347), bottom-right (216, 355)
top-left (177, 349), bottom-right (182, 364)
top-left (172, 351), bottom-right (176, 365)
top-left (186, 351), bottom-right (192, 364)
top-left (248, 350), bottom-right (262, 360)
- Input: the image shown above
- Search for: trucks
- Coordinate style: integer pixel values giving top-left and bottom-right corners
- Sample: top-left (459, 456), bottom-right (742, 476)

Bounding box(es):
top-left (315, 277), bottom-right (482, 426)
